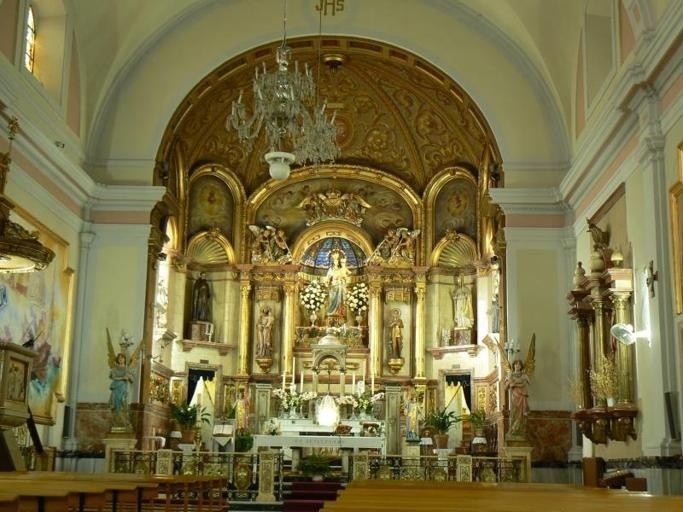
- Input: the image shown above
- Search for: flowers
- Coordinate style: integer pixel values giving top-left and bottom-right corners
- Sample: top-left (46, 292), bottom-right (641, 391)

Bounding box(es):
top-left (336, 390), bottom-right (384, 410)
top-left (273, 384), bottom-right (316, 410)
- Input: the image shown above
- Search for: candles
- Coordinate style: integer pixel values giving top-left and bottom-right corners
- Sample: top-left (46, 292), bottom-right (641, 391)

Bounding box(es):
top-left (300, 370), bottom-right (303, 394)
top-left (282, 372), bottom-right (285, 392)
top-left (292, 356), bottom-right (295, 383)
top-left (371, 374), bottom-right (374, 396)
top-left (352, 371), bottom-right (355, 396)
top-left (363, 359), bottom-right (366, 383)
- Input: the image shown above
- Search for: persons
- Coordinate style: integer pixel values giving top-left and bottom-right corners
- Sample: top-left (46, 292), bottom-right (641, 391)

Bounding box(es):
top-left (384, 309), bottom-right (404, 359)
top-left (448, 272), bottom-right (475, 331)
top-left (325, 253), bottom-right (350, 316)
top-left (254, 304), bottom-right (275, 359)
top-left (268, 229), bottom-right (285, 261)
top-left (107, 352), bottom-right (132, 428)
top-left (504, 360), bottom-right (529, 437)
top-left (397, 231), bottom-right (414, 261)
top-left (383, 228), bottom-right (399, 258)
top-left (343, 191), bottom-right (360, 220)
top-left (252, 231), bottom-right (268, 259)
top-left (308, 191), bottom-right (324, 221)
top-left (231, 390), bottom-right (251, 434)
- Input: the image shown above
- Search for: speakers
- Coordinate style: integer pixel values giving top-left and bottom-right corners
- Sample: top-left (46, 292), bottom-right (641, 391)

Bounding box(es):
top-left (664, 391), bottom-right (680, 442)
top-left (63, 405), bottom-right (70, 439)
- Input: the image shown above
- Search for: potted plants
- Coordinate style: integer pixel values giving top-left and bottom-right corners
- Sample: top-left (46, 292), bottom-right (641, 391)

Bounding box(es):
top-left (586, 354), bottom-right (621, 407)
top-left (429, 406), bottom-right (459, 449)
top-left (464, 404), bottom-right (488, 438)
top-left (295, 453), bottom-right (335, 482)
top-left (170, 397), bottom-right (212, 444)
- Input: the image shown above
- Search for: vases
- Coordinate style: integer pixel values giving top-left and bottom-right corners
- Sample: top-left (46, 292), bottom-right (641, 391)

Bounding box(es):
top-left (289, 407), bottom-right (298, 419)
top-left (359, 408), bottom-right (366, 421)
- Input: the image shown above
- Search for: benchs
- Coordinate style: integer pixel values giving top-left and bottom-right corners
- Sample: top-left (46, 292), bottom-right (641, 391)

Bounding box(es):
top-left (1, 471), bottom-right (682, 512)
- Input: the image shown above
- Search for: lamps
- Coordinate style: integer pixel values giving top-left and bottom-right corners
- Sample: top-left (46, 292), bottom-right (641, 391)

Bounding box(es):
top-left (225, 0), bottom-right (342, 181)
top-left (610, 322), bottom-right (651, 346)
top-left (0, 151), bottom-right (56, 274)
top-left (311, 366), bottom-right (347, 426)
